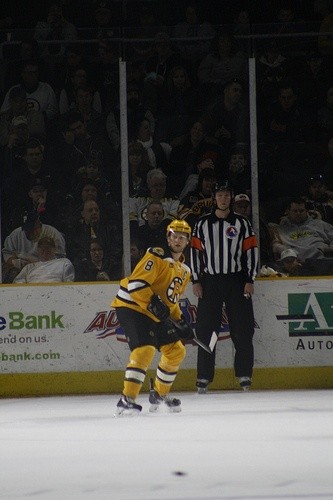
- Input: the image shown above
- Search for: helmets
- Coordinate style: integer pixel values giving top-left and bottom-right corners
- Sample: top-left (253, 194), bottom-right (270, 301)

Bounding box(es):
top-left (306, 175), bottom-right (326, 185)
top-left (211, 181), bottom-right (234, 199)
top-left (166, 219), bottom-right (192, 240)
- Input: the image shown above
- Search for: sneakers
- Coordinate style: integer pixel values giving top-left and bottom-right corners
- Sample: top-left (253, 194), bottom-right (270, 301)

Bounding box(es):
top-left (195, 379), bottom-right (209, 394)
top-left (117, 395), bottom-right (143, 417)
top-left (239, 376), bottom-right (252, 392)
top-left (148, 391), bottom-right (181, 413)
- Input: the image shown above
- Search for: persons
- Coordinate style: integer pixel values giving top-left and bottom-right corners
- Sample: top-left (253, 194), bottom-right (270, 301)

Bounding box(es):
top-left (189, 182), bottom-right (259, 388)
top-left (111, 219), bottom-right (191, 410)
top-left (0, 0), bottom-right (333, 283)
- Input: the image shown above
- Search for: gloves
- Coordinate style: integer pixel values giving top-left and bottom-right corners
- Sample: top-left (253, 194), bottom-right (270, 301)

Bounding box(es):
top-left (147, 294), bottom-right (170, 320)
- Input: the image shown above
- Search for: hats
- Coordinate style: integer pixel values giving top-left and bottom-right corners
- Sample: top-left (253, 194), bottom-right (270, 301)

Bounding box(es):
top-left (30, 179), bottom-right (47, 189)
top-left (276, 249), bottom-right (297, 262)
top-left (234, 194), bottom-right (250, 202)
top-left (21, 208), bottom-right (39, 231)
top-left (12, 116), bottom-right (28, 128)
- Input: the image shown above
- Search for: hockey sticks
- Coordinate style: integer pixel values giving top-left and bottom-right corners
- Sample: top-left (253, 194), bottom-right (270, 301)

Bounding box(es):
top-left (167, 316), bottom-right (218, 354)
